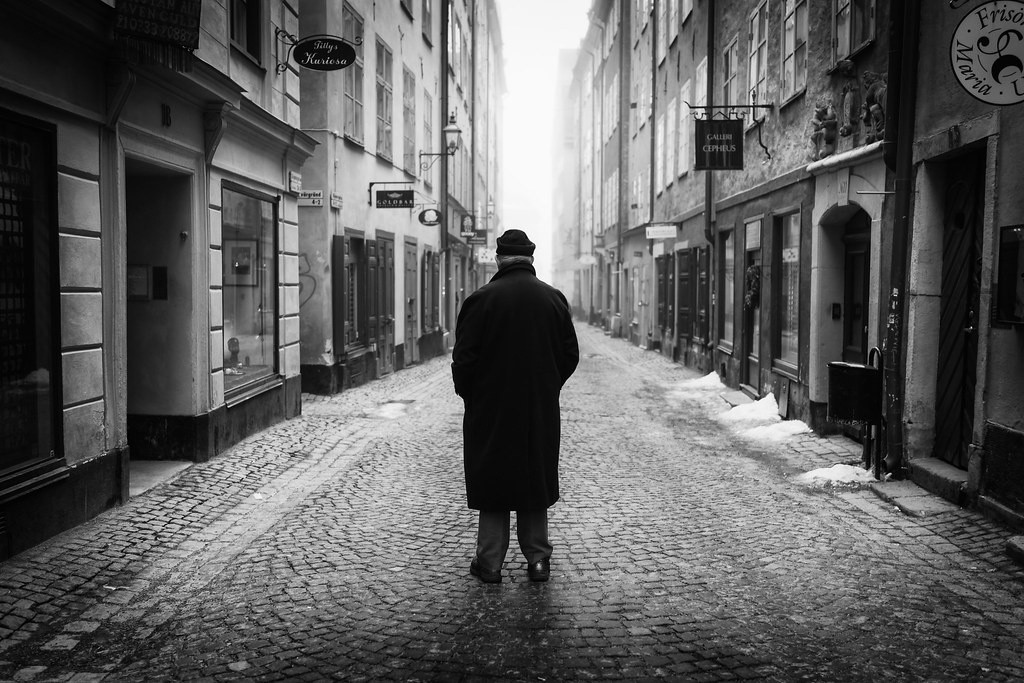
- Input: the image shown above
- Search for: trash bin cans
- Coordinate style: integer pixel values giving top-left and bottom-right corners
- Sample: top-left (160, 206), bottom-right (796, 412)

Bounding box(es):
top-left (825, 361), bottom-right (883, 428)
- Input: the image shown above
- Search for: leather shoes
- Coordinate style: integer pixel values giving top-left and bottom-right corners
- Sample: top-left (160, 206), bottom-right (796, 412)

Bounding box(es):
top-left (528, 560), bottom-right (550, 582)
top-left (470, 555), bottom-right (502, 584)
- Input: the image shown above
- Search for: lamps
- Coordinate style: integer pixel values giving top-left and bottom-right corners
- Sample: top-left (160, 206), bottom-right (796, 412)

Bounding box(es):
top-left (417, 111), bottom-right (462, 176)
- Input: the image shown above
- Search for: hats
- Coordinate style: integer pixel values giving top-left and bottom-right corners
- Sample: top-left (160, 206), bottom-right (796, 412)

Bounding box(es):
top-left (496, 229), bottom-right (536, 255)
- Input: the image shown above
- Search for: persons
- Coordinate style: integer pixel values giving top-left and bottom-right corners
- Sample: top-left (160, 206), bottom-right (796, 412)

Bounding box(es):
top-left (450, 229), bottom-right (581, 582)
